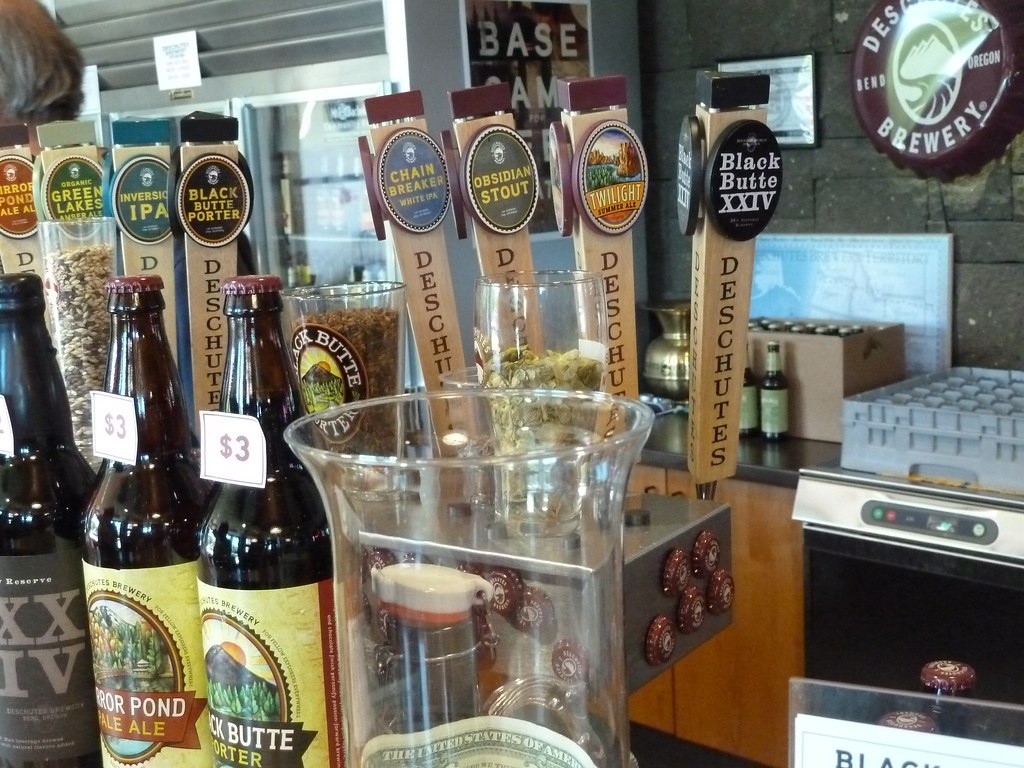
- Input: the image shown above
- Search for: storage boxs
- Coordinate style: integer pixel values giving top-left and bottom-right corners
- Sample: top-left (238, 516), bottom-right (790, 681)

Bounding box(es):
top-left (740, 323), bottom-right (905, 444)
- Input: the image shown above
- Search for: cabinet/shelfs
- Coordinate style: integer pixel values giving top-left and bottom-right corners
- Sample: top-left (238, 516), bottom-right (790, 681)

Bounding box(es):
top-left (627, 465), bottom-right (806, 768)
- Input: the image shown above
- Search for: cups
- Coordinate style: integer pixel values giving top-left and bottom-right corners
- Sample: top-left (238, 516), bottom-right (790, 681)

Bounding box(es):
top-left (472, 271), bottom-right (611, 538)
top-left (283, 385), bottom-right (656, 768)
top-left (279, 280), bottom-right (408, 457)
top-left (437, 367), bottom-right (496, 506)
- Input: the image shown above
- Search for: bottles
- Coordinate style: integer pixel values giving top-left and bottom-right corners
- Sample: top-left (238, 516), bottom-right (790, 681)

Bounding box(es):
top-left (0, 270), bottom-right (98, 768)
top-left (877, 710), bottom-right (938, 734)
top-left (920, 660), bottom-right (976, 737)
top-left (758, 341), bottom-right (790, 441)
top-left (739, 343), bottom-right (760, 439)
top-left (78, 273), bottom-right (218, 768)
top-left (199, 274), bottom-right (345, 768)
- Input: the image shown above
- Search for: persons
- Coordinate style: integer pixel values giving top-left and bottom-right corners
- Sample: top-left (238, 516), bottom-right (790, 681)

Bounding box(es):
top-left (0, 0), bottom-right (255, 427)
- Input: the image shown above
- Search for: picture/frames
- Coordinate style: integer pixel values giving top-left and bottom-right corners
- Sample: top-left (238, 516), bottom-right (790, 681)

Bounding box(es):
top-left (713, 52), bottom-right (820, 150)
